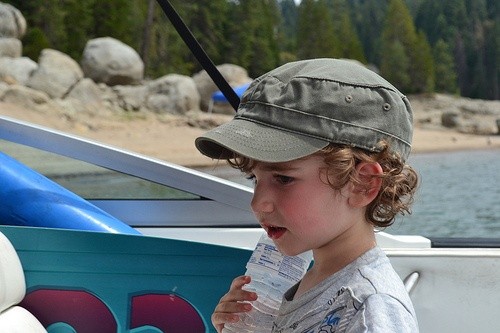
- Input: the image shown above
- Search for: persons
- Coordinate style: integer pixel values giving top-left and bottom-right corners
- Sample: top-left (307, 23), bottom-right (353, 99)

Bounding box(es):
top-left (195, 60), bottom-right (419, 333)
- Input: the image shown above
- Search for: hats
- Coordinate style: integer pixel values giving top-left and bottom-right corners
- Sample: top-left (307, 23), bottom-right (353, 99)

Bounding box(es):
top-left (194, 58), bottom-right (415, 166)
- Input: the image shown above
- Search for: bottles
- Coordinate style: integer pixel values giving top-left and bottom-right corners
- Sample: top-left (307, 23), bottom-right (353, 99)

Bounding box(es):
top-left (219, 226), bottom-right (313, 333)
